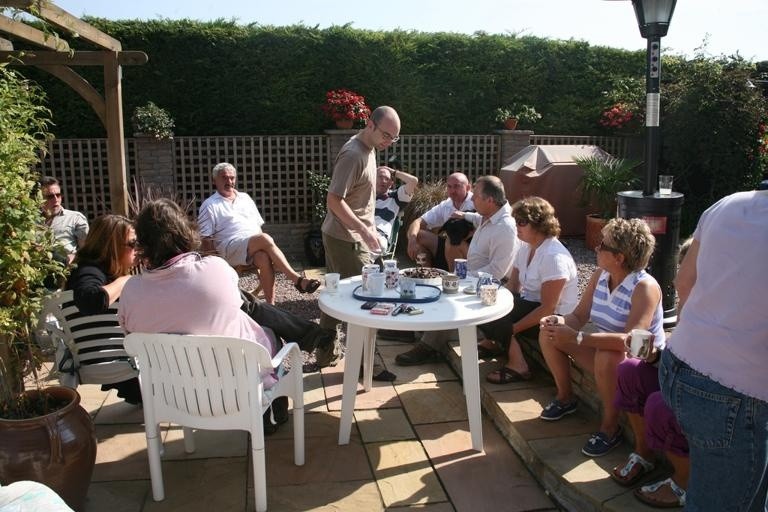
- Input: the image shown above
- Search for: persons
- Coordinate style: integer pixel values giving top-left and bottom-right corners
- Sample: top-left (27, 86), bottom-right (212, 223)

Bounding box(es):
top-left (312, 105), bottom-right (401, 381)
top-left (611, 236), bottom-right (696, 507)
top-left (118, 197), bottom-right (337, 435)
top-left (198, 163), bottom-right (321, 306)
top-left (63, 214), bottom-right (142, 404)
top-left (374, 166), bottom-right (418, 266)
top-left (477, 197), bottom-right (578, 384)
top-left (538, 218), bottom-right (668, 457)
top-left (658, 179), bottom-right (768, 512)
top-left (395, 175), bottom-right (531, 366)
top-left (40, 177), bottom-right (90, 291)
top-left (406, 172), bottom-right (477, 273)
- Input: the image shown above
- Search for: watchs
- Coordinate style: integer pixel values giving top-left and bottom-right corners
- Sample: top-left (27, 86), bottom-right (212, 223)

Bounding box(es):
top-left (576, 331), bottom-right (584, 345)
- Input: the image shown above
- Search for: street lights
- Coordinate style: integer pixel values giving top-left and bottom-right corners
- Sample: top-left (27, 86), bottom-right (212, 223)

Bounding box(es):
top-left (630, 0), bottom-right (676, 193)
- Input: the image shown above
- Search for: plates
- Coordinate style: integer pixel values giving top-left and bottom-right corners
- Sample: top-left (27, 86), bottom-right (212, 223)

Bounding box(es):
top-left (399, 267), bottom-right (449, 286)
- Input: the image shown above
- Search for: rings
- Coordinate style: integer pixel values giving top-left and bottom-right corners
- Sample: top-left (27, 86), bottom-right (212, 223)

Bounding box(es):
top-left (549, 336), bottom-right (553, 340)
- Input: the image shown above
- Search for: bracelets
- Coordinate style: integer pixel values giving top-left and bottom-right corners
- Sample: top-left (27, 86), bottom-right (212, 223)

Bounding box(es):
top-left (391, 170), bottom-right (396, 177)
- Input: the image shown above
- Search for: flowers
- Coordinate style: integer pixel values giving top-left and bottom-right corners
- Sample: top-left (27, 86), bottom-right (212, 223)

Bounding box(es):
top-left (130, 101), bottom-right (176, 142)
top-left (495, 103), bottom-right (542, 123)
top-left (597, 103), bottom-right (637, 133)
top-left (321, 89), bottom-right (372, 126)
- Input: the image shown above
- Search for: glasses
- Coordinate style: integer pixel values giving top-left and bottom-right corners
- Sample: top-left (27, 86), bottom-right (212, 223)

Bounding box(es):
top-left (377, 126), bottom-right (401, 143)
top-left (45, 193), bottom-right (61, 200)
top-left (516, 217), bottom-right (529, 227)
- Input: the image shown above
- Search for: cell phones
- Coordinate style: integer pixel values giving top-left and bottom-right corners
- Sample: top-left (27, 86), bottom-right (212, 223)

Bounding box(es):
top-left (361, 301), bottom-right (377, 310)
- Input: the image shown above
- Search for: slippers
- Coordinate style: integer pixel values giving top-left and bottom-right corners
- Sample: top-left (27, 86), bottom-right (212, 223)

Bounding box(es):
top-left (610, 451), bottom-right (655, 486)
top-left (633, 477), bottom-right (688, 509)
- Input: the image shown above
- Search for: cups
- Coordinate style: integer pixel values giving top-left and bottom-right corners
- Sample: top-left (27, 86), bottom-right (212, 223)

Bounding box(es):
top-left (442, 276), bottom-right (459, 294)
top-left (659, 176), bottom-right (673, 194)
top-left (480, 285), bottom-right (498, 306)
top-left (324, 273), bottom-right (340, 293)
top-left (624, 329), bottom-right (652, 359)
top-left (454, 259), bottom-right (467, 279)
top-left (362, 260), bottom-right (415, 299)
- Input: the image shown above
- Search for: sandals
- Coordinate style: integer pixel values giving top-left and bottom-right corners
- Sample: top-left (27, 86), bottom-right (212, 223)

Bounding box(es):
top-left (485, 365), bottom-right (532, 384)
top-left (476, 343), bottom-right (504, 359)
top-left (293, 276), bottom-right (321, 294)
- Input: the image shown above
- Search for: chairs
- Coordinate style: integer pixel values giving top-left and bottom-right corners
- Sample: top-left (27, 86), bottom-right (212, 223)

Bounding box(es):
top-left (43, 289), bottom-right (143, 385)
top-left (380, 212), bottom-right (403, 264)
top-left (232, 264), bottom-right (264, 301)
top-left (123, 331), bottom-right (305, 512)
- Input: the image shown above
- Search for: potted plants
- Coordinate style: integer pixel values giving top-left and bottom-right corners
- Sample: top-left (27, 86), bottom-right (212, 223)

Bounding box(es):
top-left (576, 157), bottom-right (648, 251)
top-left (0, 59), bottom-right (97, 512)
top-left (304, 170), bottom-right (331, 267)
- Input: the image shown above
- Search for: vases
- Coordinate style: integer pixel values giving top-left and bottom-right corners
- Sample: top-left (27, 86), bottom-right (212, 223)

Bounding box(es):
top-left (336, 119), bottom-right (353, 129)
top-left (505, 118), bottom-right (517, 130)
top-left (617, 129), bottom-right (635, 137)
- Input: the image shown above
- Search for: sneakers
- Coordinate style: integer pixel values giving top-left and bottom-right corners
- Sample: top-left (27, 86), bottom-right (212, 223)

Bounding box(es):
top-left (372, 369), bottom-right (398, 381)
top-left (580, 424), bottom-right (625, 458)
top-left (394, 340), bottom-right (444, 367)
top-left (319, 328), bottom-right (337, 350)
top-left (316, 351), bottom-right (343, 369)
top-left (539, 398), bottom-right (579, 420)
